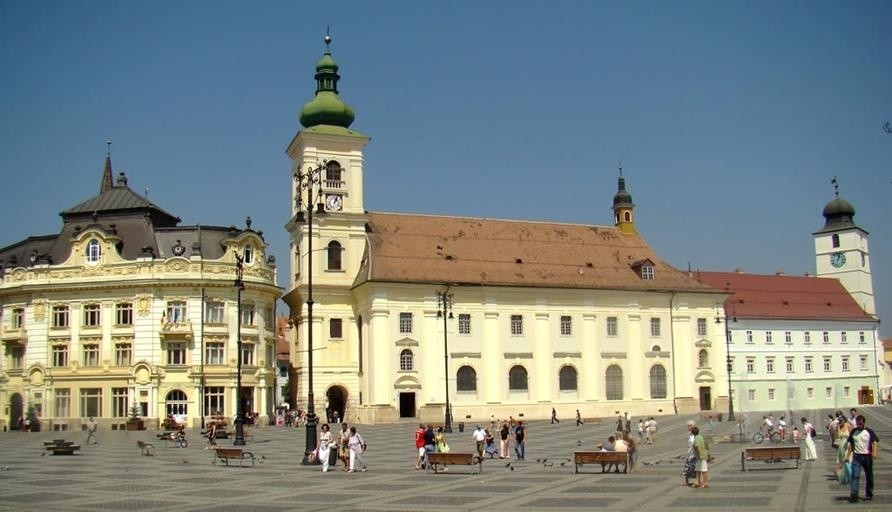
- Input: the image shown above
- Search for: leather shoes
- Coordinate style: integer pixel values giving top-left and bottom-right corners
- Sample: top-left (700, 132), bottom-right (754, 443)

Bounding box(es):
top-left (867, 496), bottom-right (872, 500)
top-left (848, 498), bottom-right (857, 502)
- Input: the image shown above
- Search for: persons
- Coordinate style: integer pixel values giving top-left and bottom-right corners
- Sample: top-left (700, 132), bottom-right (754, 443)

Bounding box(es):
top-left (550, 407), bottom-right (560, 424)
top-left (800, 417), bottom-right (818, 463)
top-left (316, 423), bottom-right (334, 473)
top-left (327, 408), bottom-right (339, 423)
top-left (432, 426), bottom-right (450, 471)
top-left (423, 423), bottom-right (436, 470)
top-left (209, 421), bottom-right (219, 449)
top-left (335, 422), bottom-right (353, 472)
top-left (688, 426), bottom-right (713, 489)
top-left (759, 416), bottom-right (775, 436)
top-left (598, 411), bottom-right (658, 473)
top-left (791, 427), bottom-right (799, 445)
top-left (348, 427), bottom-right (368, 473)
top-left (16, 415), bottom-right (33, 433)
top-left (415, 423), bottom-right (428, 469)
top-left (685, 419), bottom-right (700, 459)
top-left (285, 406), bottom-right (309, 428)
top-left (828, 407), bottom-right (880, 503)
top-left (576, 409), bottom-right (583, 426)
top-left (86, 416), bottom-right (98, 445)
top-left (777, 416), bottom-right (787, 442)
top-left (470, 414), bottom-right (528, 461)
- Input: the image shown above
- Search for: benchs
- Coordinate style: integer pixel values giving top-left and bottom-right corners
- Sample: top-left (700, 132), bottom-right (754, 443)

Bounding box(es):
top-left (739, 448), bottom-right (801, 472)
top-left (425, 452), bottom-right (482, 475)
top-left (573, 451), bottom-right (627, 474)
top-left (214, 449), bottom-right (255, 468)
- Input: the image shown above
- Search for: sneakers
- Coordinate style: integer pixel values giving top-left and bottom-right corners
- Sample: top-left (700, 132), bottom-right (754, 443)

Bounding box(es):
top-left (362, 467), bottom-right (368, 472)
top-left (348, 470), bottom-right (354, 473)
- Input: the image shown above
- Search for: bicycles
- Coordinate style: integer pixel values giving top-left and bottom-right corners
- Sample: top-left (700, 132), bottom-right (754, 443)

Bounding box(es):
top-left (754, 426), bottom-right (783, 444)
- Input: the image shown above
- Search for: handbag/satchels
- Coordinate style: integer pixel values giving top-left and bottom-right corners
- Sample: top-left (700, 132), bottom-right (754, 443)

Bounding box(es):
top-left (811, 428), bottom-right (816, 437)
top-left (328, 432), bottom-right (336, 448)
top-left (357, 434), bottom-right (366, 450)
top-left (440, 443), bottom-right (449, 453)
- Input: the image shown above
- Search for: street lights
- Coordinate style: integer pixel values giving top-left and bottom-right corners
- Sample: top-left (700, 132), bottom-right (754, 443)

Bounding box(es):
top-left (294, 158), bottom-right (331, 466)
top-left (231, 249), bottom-right (247, 449)
top-left (435, 283), bottom-right (458, 434)
top-left (715, 297), bottom-right (738, 423)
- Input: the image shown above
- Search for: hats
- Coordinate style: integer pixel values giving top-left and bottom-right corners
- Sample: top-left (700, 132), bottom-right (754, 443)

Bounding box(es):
top-left (837, 415), bottom-right (845, 421)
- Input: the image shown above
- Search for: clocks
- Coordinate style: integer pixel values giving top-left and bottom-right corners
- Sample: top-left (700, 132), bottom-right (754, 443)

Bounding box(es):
top-left (830, 252), bottom-right (847, 269)
top-left (326, 194), bottom-right (345, 213)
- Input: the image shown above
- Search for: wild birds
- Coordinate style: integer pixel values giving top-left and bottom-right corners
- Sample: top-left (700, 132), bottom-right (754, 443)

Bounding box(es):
top-left (5, 466), bottom-right (9, 471)
top-left (567, 458), bottom-right (571, 462)
top-left (642, 463), bottom-right (650, 465)
top-left (41, 453), bottom-right (46, 456)
top-left (537, 458), bottom-right (540, 463)
top-left (257, 458), bottom-right (264, 465)
top-left (182, 459), bottom-right (188, 464)
top-left (221, 459), bottom-right (226, 463)
top-left (511, 467), bottom-right (514, 471)
top-left (561, 462), bottom-right (565, 466)
top-left (505, 463), bottom-right (511, 468)
top-left (682, 456), bottom-right (686, 459)
top-left (542, 459), bottom-right (547, 463)
top-left (262, 456), bottom-right (266, 459)
top-left (676, 455), bottom-right (681, 459)
